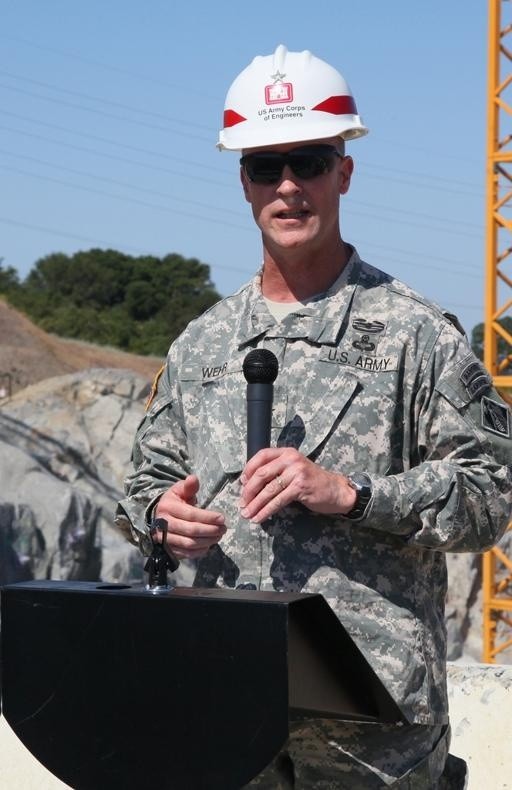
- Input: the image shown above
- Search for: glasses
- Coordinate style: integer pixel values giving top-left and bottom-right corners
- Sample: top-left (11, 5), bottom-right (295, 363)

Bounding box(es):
top-left (239, 143), bottom-right (344, 184)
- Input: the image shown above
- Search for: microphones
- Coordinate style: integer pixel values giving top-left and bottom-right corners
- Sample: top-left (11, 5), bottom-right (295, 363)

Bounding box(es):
top-left (242, 349), bottom-right (278, 521)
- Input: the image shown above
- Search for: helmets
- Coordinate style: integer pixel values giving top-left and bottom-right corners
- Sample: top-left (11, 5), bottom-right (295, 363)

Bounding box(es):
top-left (216, 44), bottom-right (370, 151)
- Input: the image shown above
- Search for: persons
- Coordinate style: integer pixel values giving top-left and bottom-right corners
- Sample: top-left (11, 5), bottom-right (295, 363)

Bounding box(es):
top-left (113, 130), bottom-right (512, 789)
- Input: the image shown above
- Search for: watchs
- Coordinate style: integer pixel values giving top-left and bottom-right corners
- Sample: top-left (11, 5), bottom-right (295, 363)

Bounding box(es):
top-left (347, 471), bottom-right (372, 519)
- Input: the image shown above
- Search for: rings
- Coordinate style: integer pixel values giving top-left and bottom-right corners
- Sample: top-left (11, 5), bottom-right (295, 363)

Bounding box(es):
top-left (276, 476), bottom-right (286, 490)
